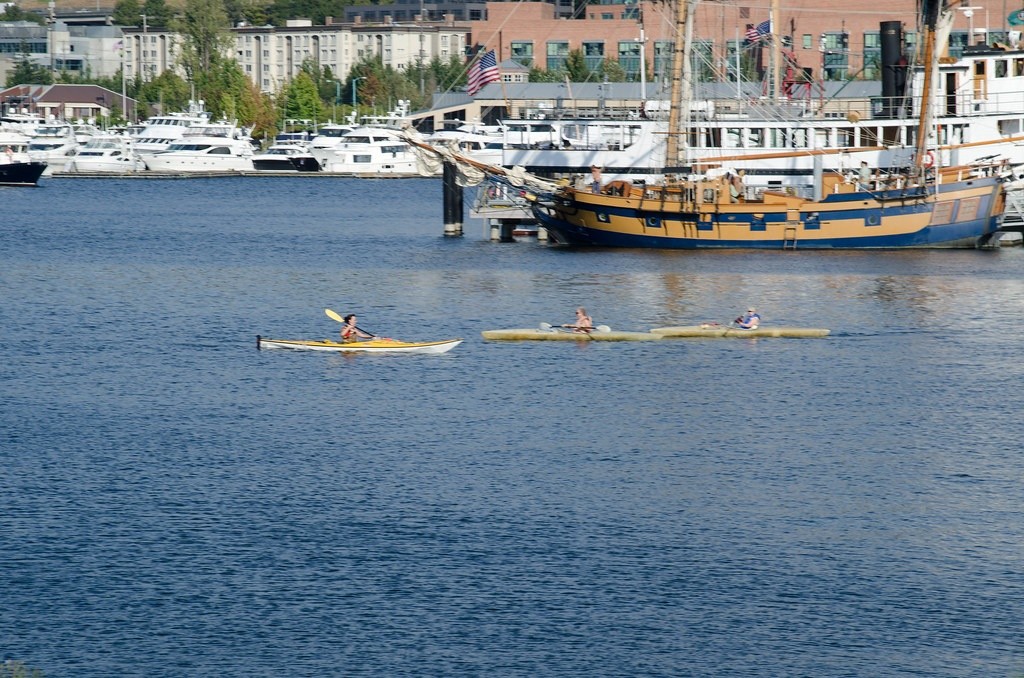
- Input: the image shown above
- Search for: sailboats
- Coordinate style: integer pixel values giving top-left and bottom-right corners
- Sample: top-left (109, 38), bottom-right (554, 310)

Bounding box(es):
top-left (390, 0), bottom-right (1024, 250)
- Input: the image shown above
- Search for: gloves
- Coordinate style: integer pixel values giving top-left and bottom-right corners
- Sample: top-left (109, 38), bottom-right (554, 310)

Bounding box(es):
top-left (735, 317), bottom-right (742, 323)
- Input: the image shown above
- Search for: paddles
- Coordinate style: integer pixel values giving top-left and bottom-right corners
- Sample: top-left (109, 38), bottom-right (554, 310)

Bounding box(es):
top-left (722, 314), bottom-right (745, 337)
top-left (540, 322), bottom-right (612, 334)
top-left (324, 308), bottom-right (375, 337)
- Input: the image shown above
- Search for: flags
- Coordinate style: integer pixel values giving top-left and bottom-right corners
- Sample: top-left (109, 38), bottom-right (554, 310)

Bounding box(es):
top-left (745, 20), bottom-right (770, 42)
top-left (467, 49), bottom-right (501, 96)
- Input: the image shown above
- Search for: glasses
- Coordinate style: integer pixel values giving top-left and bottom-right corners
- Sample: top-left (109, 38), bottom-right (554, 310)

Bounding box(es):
top-left (748, 311), bottom-right (754, 312)
top-left (576, 312), bottom-right (582, 314)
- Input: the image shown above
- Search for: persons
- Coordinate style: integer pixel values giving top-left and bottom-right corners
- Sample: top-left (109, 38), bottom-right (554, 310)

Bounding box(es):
top-left (341, 314), bottom-right (372, 344)
top-left (591, 165), bottom-right (602, 194)
top-left (859, 161), bottom-right (871, 192)
top-left (735, 308), bottom-right (760, 330)
top-left (730, 169), bottom-right (745, 203)
top-left (562, 307), bottom-right (592, 334)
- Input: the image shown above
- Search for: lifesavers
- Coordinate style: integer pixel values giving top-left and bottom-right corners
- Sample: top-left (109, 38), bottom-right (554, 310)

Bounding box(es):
top-left (924, 151), bottom-right (934, 169)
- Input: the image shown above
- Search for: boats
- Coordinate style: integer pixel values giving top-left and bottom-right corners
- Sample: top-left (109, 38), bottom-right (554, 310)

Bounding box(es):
top-left (483, 328), bottom-right (664, 342)
top-left (256, 332), bottom-right (465, 355)
top-left (0, 39), bottom-right (1024, 245)
top-left (648, 321), bottom-right (832, 339)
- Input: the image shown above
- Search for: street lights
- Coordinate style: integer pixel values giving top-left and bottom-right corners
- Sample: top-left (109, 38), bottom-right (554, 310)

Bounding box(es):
top-left (326, 80), bottom-right (340, 107)
top-left (353, 77), bottom-right (368, 108)
